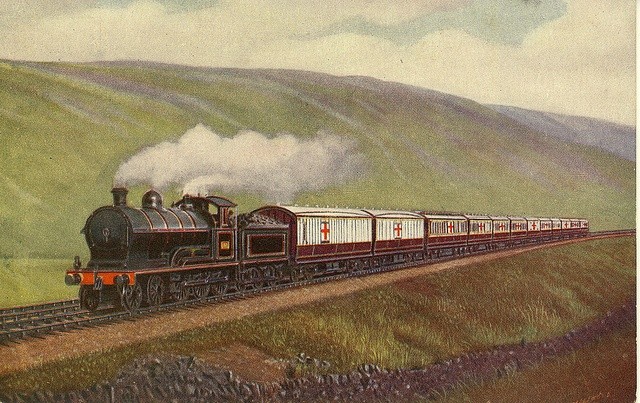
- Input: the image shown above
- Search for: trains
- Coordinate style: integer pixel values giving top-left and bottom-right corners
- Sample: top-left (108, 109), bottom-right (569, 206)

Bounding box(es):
top-left (65, 188), bottom-right (589, 312)
